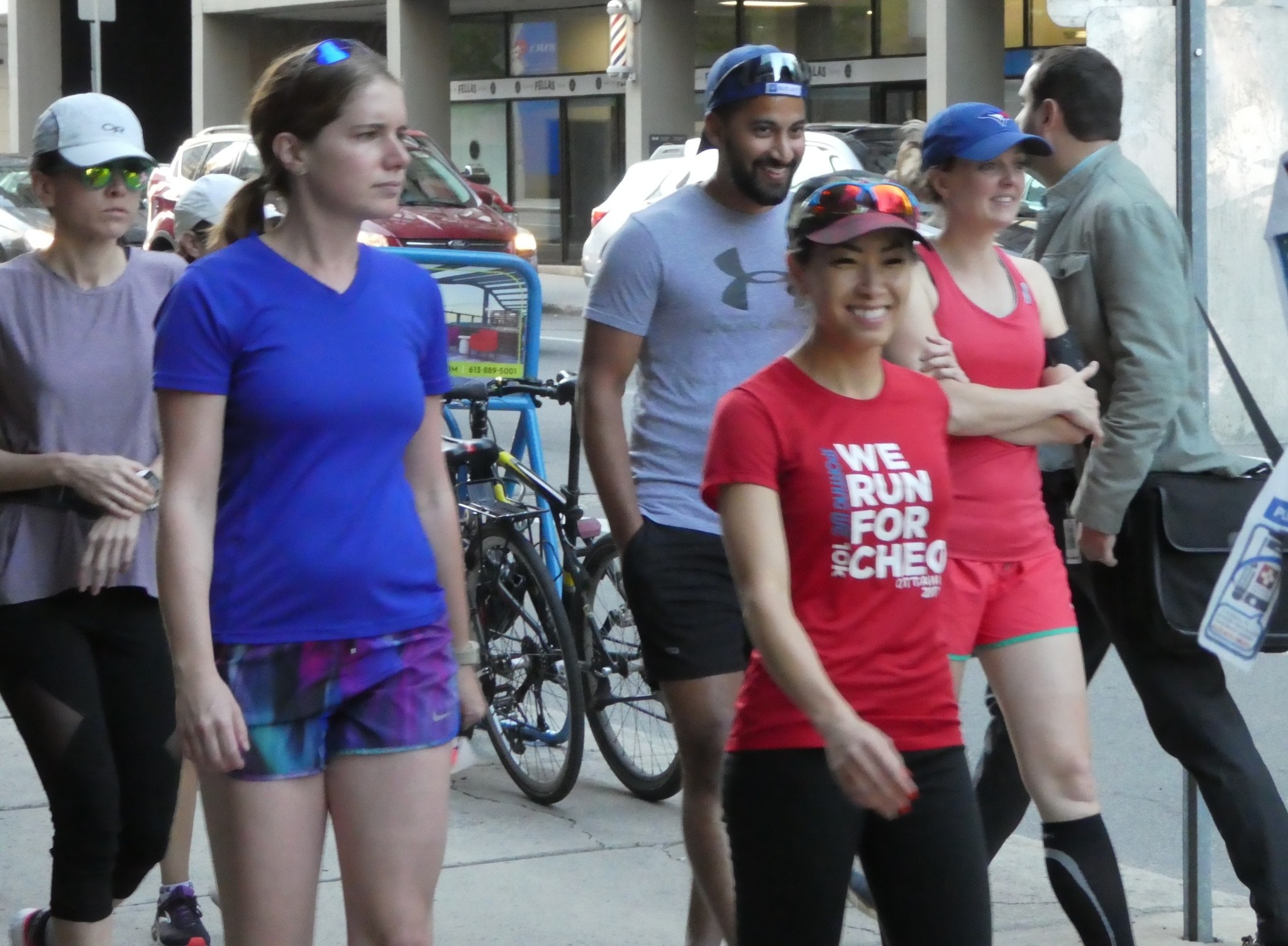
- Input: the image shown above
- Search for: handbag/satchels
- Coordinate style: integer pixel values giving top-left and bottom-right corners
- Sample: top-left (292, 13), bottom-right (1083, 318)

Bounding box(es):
top-left (1130, 464), bottom-right (1288, 657)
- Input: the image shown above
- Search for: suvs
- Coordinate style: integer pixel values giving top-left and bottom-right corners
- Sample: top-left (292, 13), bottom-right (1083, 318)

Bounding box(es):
top-left (143, 124), bottom-right (539, 315)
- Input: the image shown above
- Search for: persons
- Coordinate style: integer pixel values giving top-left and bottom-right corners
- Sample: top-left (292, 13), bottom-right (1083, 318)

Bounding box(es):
top-left (577, 44), bottom-right (822, 946)
top-left (837, 44), bottom-right (1288, 946)
top-left (0, 91), bottom-right (203, 946)
top-left (881, 102), bottom-right (1137, 946)
top-left (148, 40), bottom-right (493, 946)
top-left (146, 169), bottom-right (275, 946)
top-left (707, 168), bottom-right (998, 946)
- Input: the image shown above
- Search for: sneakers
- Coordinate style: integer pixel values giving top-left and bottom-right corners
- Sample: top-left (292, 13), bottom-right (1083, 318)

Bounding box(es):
top-left (151, 889), bottom-right (212, 946)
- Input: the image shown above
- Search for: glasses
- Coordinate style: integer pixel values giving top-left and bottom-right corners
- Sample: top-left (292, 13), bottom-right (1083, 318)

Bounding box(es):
top-left (703, 53), bottom-right (811, 101)
top-left (300, 39), bottom-right (352, 64)
top-left (794, 183), bottom-right (919, 219)
top-left (74, 168), bottom-right (148, 190)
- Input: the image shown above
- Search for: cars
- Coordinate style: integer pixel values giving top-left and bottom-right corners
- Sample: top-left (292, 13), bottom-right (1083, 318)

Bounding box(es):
top-left (0, 172), bottom-right (59, 263)
top-left (578, 121), bottom-right (1048, 313)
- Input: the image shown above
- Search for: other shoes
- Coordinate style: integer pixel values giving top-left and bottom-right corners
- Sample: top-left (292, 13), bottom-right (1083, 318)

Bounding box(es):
top-left (846, 870), bottom-right (880, 918)
top-left (9, 907), bottom-right (50, 946)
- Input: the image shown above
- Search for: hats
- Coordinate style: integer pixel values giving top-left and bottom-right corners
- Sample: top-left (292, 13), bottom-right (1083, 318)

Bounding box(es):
top-left (920, 101), bottom-right (1056, 168)
top-left (173, 173), bottom-right (286, 234)
top-left (786, 168), bottom-right (933, 255)
top-left (695, 44), bottom-right (809, 155)
top-left (30, 93), bottom-right (160, 168)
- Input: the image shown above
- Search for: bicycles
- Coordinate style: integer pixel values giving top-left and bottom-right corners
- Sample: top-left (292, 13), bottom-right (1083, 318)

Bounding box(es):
top-left (440, 368), bottom-right (688, 807)
